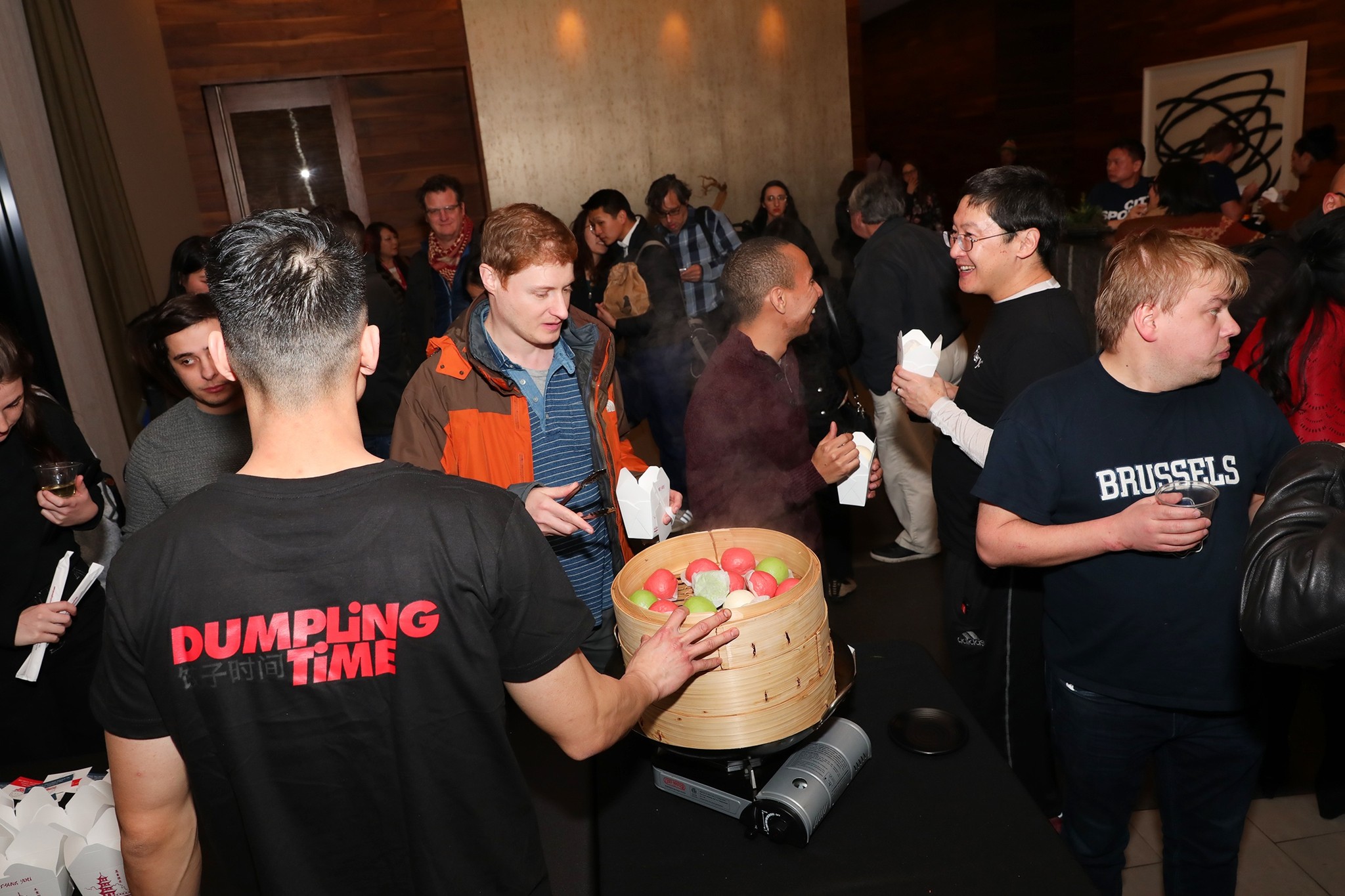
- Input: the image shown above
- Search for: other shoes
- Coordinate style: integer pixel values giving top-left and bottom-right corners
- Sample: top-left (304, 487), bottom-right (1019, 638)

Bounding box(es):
top-left (869, 539), bottom-right (934, 564)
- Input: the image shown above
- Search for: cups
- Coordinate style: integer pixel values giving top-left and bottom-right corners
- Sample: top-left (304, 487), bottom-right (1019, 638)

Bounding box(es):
top-left (32, 461), bottom-right (81, 499)
top-left (1154, 479), bottom-right (1220, 540)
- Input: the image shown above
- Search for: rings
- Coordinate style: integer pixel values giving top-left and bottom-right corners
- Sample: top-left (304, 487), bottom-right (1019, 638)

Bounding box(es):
top-left (895, 387), bottom-right (901, 394)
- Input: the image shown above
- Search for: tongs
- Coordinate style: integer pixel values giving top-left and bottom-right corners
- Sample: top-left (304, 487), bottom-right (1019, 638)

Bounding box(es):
top-left (559, 468), bottom-right (618, 521)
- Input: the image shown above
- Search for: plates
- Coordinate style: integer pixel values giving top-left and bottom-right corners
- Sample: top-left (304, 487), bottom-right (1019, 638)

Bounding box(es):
top-left (887, 706), bottom-right (971, 757)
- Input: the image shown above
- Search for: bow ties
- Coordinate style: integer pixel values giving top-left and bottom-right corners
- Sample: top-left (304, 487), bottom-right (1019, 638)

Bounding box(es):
top-left (617, 241), bottom-right (629, 258)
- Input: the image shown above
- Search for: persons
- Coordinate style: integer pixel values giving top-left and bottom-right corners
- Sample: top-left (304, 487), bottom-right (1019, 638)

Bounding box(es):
top-left (94, 209), bottom-right (738, 896)
top-left (128, 235), bottom-right (211, 417)
top-left (1001, 140), bottom-right (1017, 165)
top-left (305, 204), bottom-right (398, 456)
top-left (0, 334), bottom-right (124, 782)
top-left (899, 160), bottom-right (944, 233)
top-left (569, 188), bottom-right (694, 533)
top-left (124, 293), bottom-right (252, 538)
top-left (1228, 165), bottom-right (1345, 447)
top-left (681, 237), bottom-right (883, 556)
top-left (845, 172), bottom-right (967, 562)
top-left (404, 174), bottom-right (482, 374)
top-left (740, 181), bottom-right (830, 281)
top-left (645, 173), bottom-right (742, 355)
top-left (390, 203), bottom-right (683, 896)
top-left (1092, 125), bottom-right (1342, 247)
top-left (970, 227), bottom-right (1300, 896)
top-left (361, 219), bottom-right (410, 305)
top-left (891, 165), bottom-right (1094, 802)
top-left (834, 170), bottom-right (867, 262)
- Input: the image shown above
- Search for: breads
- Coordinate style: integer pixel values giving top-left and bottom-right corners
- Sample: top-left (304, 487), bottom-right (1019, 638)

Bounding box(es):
top-left (627, 548), bottom-right (801, 614)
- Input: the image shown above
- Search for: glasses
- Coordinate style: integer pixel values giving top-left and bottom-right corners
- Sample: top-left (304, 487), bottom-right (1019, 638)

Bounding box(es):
top-left (657, 202), bottom-right (685, 219)
top-left (942, 229), bottom-right (1025, 251)
top-left (425, 203), bottom-right (459, 218)
top-left (764, 195), bottom-right (788, 204)
top-left (585, 224), bottom-right (595, 235)
